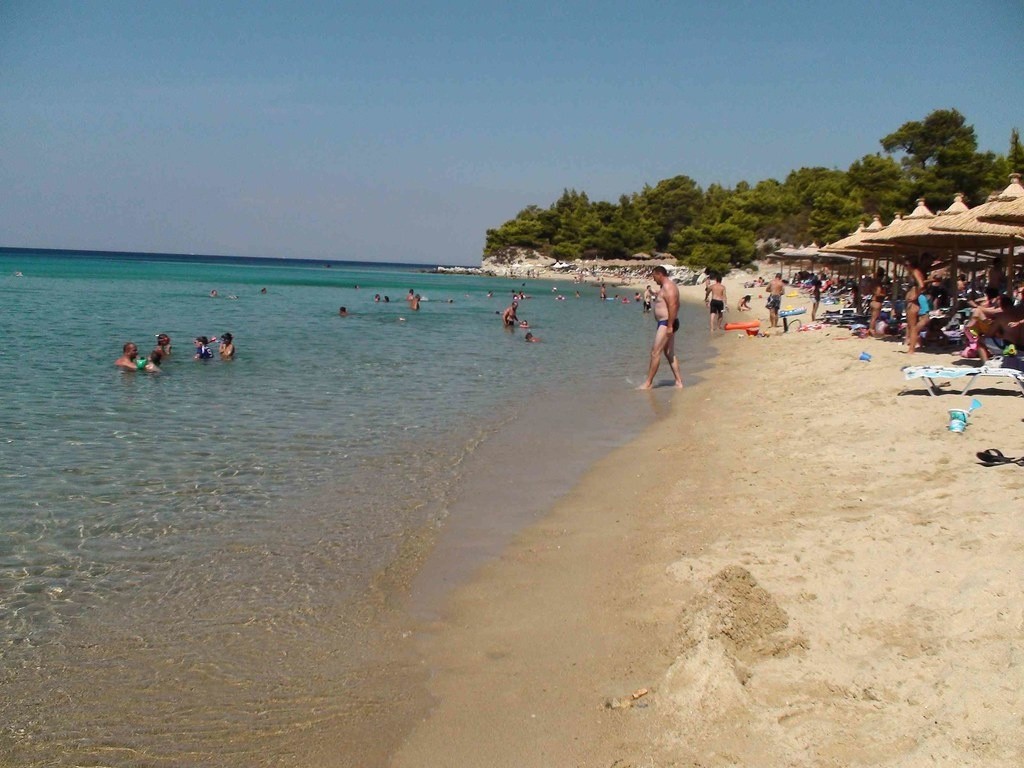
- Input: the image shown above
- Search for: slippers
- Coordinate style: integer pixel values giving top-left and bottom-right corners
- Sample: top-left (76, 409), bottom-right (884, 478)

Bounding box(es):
top-left (976, 447), bottom-right (1016, 463)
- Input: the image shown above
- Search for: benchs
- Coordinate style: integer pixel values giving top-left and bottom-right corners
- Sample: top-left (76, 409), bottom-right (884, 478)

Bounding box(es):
top-left (900, 364), bottom-right (1024, 397)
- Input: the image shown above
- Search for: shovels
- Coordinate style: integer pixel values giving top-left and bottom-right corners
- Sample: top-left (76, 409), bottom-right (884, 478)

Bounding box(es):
top-left (968, 398), bottom-right (982, 414)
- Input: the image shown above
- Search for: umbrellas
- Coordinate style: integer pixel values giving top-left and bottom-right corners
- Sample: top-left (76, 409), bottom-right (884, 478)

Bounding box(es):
top-left (762, 172), bottom-right (1024, 312)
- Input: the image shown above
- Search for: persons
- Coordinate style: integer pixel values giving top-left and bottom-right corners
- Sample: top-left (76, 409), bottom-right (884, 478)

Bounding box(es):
top-left (635, 265), bottom-right (684, 390)
top-left (736, 251), bottom-right (1024, 366)
top-left (704, 275), bottom-right (728, 333)
top-left (484, 265), bottom-right (662, 344)
top-left (113, 284), bottom-right (476, 378)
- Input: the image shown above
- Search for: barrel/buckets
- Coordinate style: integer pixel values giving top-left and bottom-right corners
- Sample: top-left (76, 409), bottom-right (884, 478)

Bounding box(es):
top-left (948, 420), bottom-right (965, 433)
top-left (859, 352), bottom-right (871, 360)
top-left (948, 410), bottom-right (971, 423)
